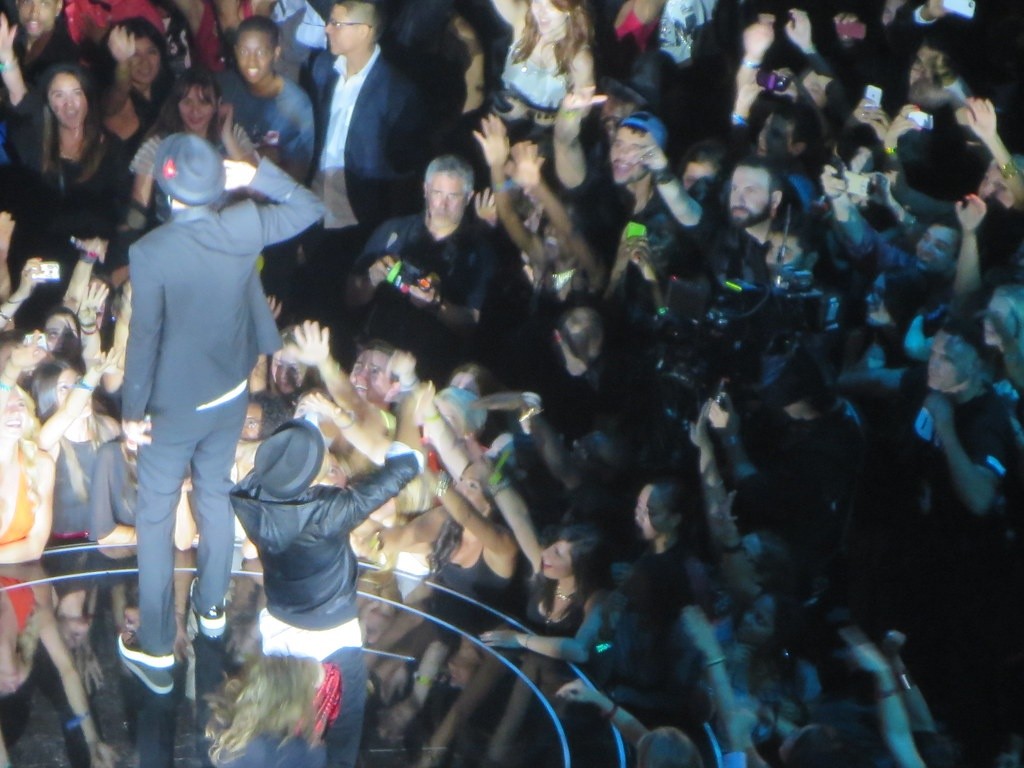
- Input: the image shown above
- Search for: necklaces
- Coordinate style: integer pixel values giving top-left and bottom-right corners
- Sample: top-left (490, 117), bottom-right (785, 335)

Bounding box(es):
top-left (556, 584), bottom-right (574, 601)
top-left (544, 601), bottom-right (573, 624)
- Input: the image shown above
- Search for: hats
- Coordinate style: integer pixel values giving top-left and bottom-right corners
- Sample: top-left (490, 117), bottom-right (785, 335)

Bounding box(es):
top-left (620, 109), bottom-right (666, 146)
top-left (252, 418), bottom-right (326, 498)
top-left (157, 131), bottom-right (227, 204)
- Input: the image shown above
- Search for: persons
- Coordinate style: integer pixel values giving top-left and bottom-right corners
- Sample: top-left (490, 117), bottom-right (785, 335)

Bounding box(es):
top-left (121, 132), bottom-right (327, 668)
top-left (229, 392), bottom-right (424, 768)
top-left (201, 655), bottom-right (327, 768)
top-left (354, 0), bottom-right (1024, 768)
top-left (0, 562), bottom-right (576, 768)
top-left (0, 0), bottom-right (424, 575)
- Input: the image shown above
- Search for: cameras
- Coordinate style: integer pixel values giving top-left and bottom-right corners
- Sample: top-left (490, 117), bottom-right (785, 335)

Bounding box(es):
top-left (757, 68), bottom-right (789, 92)
top-left (387, 260), bottom-right (423, 295)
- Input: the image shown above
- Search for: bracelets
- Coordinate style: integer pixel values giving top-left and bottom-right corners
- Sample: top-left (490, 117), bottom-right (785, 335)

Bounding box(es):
top-left (79, 248), bottom-right (98, 264)
top-left (705, 656), bottom-right (727, 667)
top-left (0, 310), bottom-right (14, 322)
top-left (0, 383), bottom-right (16, 391)
top-left (66, 709), bottom-right (90, 731)
top-left (486, 449), bottom-right (512, 496)
top-left (72, 378), bottom-right (95, 391)
top-left (80, 322), bottom-right (98, 334)
top-left (434, 468), bottom-right (450, 497)
top-left (335, 410), bottom-right (356, 430)
top-left (526, 634), bottom-right (533, 649)
top-left (601, 702), bottom-right (619, 721)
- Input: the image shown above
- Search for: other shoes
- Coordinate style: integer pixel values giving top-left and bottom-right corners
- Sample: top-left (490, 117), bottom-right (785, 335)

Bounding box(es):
top-left (191, 575), bottom-right (227, 637)
top-left (116, 628), bottom-right (173, 669)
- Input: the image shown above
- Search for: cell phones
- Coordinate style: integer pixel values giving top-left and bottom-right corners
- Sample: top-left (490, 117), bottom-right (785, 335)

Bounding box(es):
top-left (627, 221), bottom-right (647, 245)
top-left (829, 158), bottom-right (844, 181)
top-left (908, 109), bottom-right (933, 130)
top-left (24, 333), bottom-right (46, 351)
top-left (31, 261), bottom-right (61, 280)
top-left (863, 85), bottom-right (882, 108)
